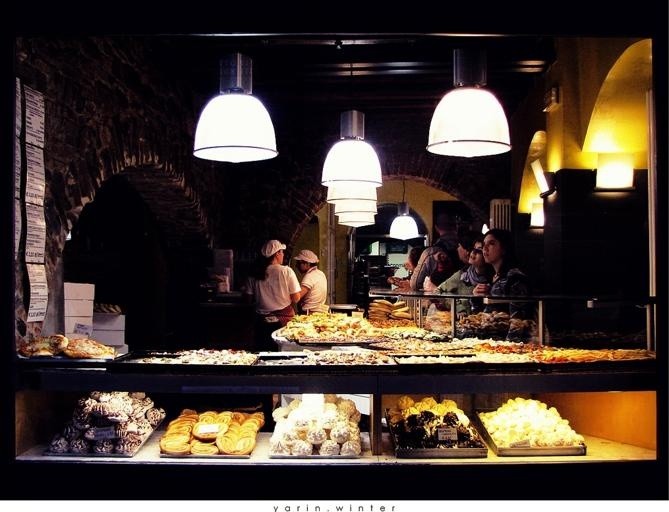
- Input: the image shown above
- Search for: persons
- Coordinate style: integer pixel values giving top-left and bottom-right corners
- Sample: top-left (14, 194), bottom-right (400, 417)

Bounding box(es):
top-left (387, 209), bottom-right (534, 318)
top-left (293, 250), bottom-right (328, 313)
top-left (241, 239), bottom-right (304, 351)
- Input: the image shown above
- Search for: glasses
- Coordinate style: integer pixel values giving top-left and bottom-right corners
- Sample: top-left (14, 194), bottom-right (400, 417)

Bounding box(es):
top-left (471, 247), bottom-right (482, 255)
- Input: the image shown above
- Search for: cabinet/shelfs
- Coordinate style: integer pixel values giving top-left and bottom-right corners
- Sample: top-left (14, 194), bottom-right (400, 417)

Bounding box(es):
top-left (2, 356), bottom-right (668, 499)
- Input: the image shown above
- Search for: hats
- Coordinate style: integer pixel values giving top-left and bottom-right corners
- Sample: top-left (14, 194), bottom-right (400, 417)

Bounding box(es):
top-left (293, 249), bottom-right (320, 264)
top-left (261, 239), bottom-right (287, 258)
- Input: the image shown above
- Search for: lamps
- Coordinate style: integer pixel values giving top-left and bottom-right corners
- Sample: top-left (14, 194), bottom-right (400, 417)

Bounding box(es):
top-left (425, 47), bottom-right (513, 157)
top-left (192, 53), bottom-right (279, 163)
top-left (320, 110), bottom-right (383, 227)
top-left (385, 176), bottom-right (424, 241)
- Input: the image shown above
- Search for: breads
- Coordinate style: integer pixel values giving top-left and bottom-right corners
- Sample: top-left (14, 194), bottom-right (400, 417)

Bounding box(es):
top-left (50, 391), bottom-right (166, 456)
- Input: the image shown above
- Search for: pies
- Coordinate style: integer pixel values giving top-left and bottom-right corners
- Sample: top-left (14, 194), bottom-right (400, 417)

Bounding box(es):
top-left (21, 333), bottom-right (120, 359)
top-left (368, 299), bottom-right (412, 320)
